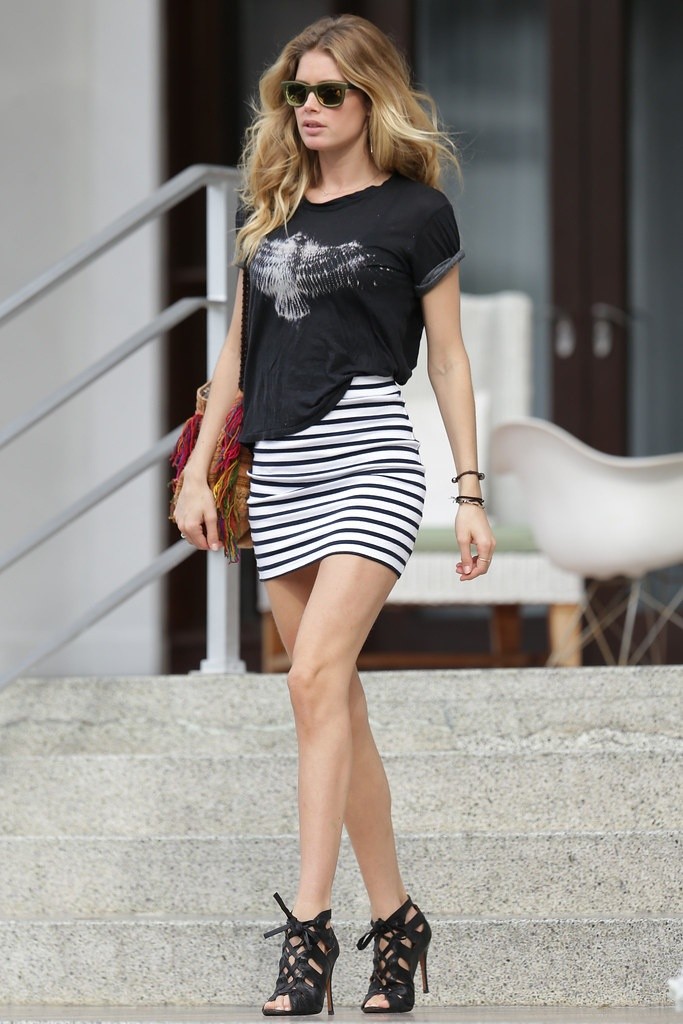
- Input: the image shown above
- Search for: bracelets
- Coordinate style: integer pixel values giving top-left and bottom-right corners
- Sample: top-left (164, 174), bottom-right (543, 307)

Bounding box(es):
top-left (451, 470), bottom-right (486, 483)
top-left (450, 494), bottom-right (487, 509)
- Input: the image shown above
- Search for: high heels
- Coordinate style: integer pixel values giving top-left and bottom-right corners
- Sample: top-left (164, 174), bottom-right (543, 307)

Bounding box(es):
top-left (261, 891), bottom-right (341, 1017)
top-left (355, 894), bottom-right (432, 1014)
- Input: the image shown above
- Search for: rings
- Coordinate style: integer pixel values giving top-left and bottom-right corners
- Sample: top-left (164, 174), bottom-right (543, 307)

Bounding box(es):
top-left (180, 533), bottom-right (187, 539)
top-left (476, 557), bottom-right (491, 565)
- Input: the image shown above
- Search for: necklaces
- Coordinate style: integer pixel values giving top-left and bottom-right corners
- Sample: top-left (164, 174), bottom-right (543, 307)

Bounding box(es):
top-left (318, 171), bottom-right (382, 200)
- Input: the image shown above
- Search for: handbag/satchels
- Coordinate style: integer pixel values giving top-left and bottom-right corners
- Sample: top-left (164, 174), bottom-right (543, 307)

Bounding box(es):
top-left (169, 381), bottom-right (253, 564)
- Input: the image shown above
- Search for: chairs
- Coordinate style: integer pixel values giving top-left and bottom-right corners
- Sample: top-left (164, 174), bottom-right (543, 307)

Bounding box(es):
top-left (486, 417), bottom-right (682, 667)
top-left (256, 288), bottom-right (586, 668)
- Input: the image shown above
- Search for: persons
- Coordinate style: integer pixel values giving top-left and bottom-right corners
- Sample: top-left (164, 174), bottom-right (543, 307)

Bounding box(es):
top-left (170, 9), bottom-right (496, 1015)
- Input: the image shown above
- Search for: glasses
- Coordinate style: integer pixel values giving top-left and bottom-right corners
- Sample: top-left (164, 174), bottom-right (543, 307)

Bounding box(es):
top-left (280, 81), bottom-right (359, 109)
